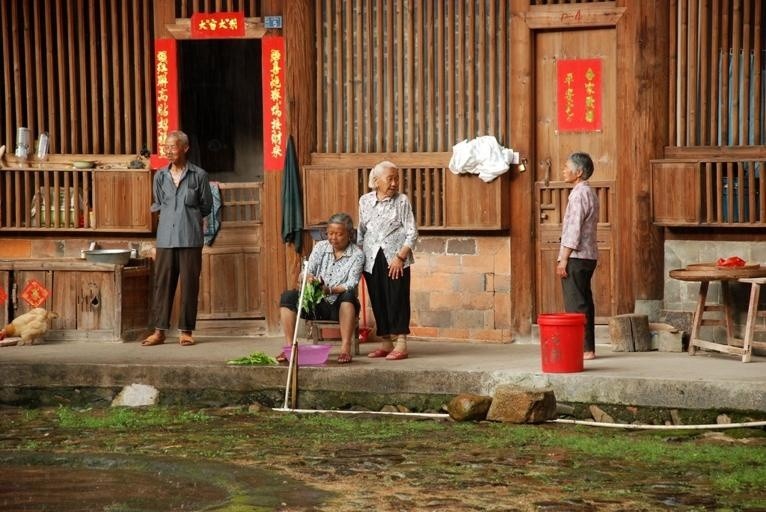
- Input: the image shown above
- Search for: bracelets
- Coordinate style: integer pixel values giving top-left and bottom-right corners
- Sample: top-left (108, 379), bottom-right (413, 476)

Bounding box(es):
top-left (327, 287), bottom-right (332, 296)
top-left (396, 252), bottom-right (406, 263)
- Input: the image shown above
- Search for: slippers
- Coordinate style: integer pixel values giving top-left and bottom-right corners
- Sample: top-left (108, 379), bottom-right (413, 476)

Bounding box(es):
top-left (338, 354), bottom-right (353, 363)
top-left (141, 336), bottom-right (164, 346)
top-left (368, 349), bottom-right (389, 358)
top-left (276, 352), bottom-right (287, 363)
top-left (386, 351), bottom-right (408, 360)
top-left (179, 336), bottom-right (195, 345)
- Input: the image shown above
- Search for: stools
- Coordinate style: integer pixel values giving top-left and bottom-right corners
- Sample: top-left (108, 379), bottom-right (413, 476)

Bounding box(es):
top-left (311, 318), bottom-right (360, 356)
top-left (688, 278), bottom-right (765, 362)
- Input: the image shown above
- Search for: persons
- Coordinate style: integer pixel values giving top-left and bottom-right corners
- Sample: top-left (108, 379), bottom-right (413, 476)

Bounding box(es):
top-left (356, 161), bottom-right (417, 361)
top-left (557, 152), bottom-right (599, 360)
top-left (140, 130), bottom-right (213, 346)
top-left (276, 213), bottom-right (364, 365)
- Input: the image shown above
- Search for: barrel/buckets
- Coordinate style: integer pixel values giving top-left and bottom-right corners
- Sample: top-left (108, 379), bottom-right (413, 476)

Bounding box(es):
top-left (536, 314), bottom-right (587, 373)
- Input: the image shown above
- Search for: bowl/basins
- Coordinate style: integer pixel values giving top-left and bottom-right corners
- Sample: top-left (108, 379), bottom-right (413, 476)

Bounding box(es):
top-left (72, 160), bottom-right (93, 168)
top-left (282, 344), bottom-right (332, 367)
top-left (81, 248), bottom-right (131, 266)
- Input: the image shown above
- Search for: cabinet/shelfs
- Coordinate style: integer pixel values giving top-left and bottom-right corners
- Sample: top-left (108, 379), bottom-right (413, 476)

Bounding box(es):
top-left (0, 259), bottom-right (150, 341)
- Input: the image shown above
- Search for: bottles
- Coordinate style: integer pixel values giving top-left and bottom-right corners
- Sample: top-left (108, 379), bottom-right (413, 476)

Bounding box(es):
top-left (16, 127), bottom-right (30, 169)
top-left (36, 132), bottom-right (48, 162)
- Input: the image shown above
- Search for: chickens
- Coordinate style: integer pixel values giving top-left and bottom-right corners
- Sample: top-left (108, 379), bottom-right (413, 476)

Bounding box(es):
top-left (0, 307), bottom-right (60, 346)
